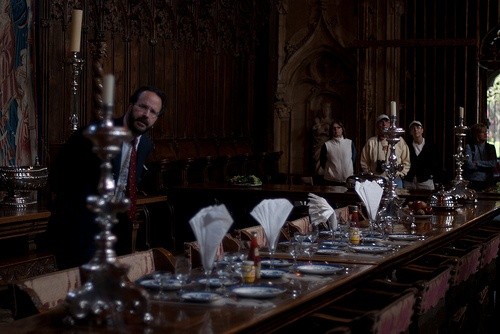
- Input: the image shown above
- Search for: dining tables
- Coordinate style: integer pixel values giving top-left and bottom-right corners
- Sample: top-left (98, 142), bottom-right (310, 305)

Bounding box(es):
top-left (230, 197), bottom-right (500, 270)
top-left (0, 256), bottom-right (380, 334)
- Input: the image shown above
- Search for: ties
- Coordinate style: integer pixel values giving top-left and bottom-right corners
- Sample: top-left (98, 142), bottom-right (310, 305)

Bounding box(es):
top-left (128, 138), bottom-right (136, 218)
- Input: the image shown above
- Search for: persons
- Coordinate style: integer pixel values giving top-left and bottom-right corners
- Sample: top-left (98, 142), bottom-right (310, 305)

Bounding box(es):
top-left (43, 85), bottom-right (167, 271)
top-left (320, 115), bottom-right (498, 194)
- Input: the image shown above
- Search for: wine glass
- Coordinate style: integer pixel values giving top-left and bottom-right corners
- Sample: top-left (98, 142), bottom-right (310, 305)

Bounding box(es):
top-left (175, 244), bottom-right (193, 303)
top-left (199, 244), bottom-right (234, 297)
top-left (329, 218), bottom-right (338, 246)
top-left (263, 228), bottom-right (281, 270)
top-left (360, 203), bottom-right (393, 245)
top-left (289, 222), bottom-right (319, 275)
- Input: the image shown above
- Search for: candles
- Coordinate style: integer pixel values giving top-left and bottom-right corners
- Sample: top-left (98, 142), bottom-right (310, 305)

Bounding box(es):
top-left (69, 9), bottom-right (84, 53)
top-left (391, 100), bottom-right (396, 116)
top-left (103, 72), bottom-right (117, 105)
top-left (459, 106), bottom-right (465, 119)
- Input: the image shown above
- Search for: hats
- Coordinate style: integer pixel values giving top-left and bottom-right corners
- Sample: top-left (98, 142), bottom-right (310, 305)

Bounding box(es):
top-left (375, 115), bottom-right (389, 124)
top-left (408, 121), bottom-right (422, 128)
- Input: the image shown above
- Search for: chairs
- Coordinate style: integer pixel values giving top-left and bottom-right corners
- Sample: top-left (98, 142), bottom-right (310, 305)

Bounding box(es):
top-left (7, 201), bottom-right (500, 334)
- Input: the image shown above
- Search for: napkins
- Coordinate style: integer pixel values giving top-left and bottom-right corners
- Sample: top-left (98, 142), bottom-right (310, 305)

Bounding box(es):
top-left (248, 197), bottom-right (294, 249)
top-left (188, 203), bottom-right (234, 275)
top-left (306, 191), bottom-right (339, 232)
top-left (354, 178), bottom-right (384, 221)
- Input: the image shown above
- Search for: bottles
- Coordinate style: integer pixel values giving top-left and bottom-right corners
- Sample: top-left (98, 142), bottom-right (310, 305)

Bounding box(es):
top-left (350, 205), bottom-right (359, 227)
top-left (241, 231), bottom-right (264, 283)
top-left (351, 227), bottom-right (360, 246)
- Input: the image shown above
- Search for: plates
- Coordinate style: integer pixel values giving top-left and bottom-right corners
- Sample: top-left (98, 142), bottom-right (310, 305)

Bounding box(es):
top-left (198, 276), bottom-right (239, 286)
top-left (182, 291), bottom-right (222, 302)
top-left (260, 259), bottom-right (345, 276)
top-left (229, 283), bottom-right (287, 299)
top-left (317, 231), bottom-right (421, 256)
top-left (279, 241), bottom-right (318, 247)
top-left (136, 273), bottom-right (193, 289)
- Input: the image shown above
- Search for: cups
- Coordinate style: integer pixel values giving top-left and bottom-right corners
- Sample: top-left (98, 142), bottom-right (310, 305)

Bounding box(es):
top-left (232, 252), bottom-right (248, 275)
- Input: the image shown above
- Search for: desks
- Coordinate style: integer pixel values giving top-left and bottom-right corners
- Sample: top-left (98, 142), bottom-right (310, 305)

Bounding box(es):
top-left (168, 180), bottom-right (441, 213)
top-left (0, 193), bottom-right (180, 309)
